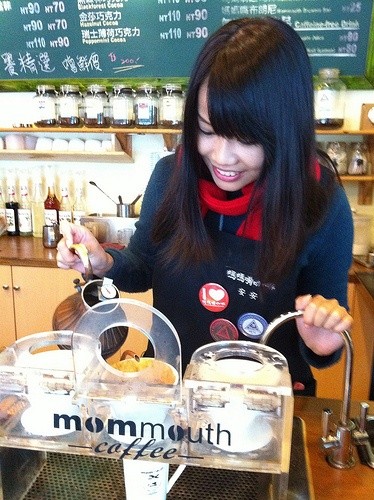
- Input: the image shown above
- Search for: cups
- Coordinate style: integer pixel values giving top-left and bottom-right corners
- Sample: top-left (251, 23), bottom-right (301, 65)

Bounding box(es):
top-left (327, 141), bottom-right (370, 175)
top-left (43, 225), bottom-right (60, 248)
top-left (116, 204), bottom-right (135, 217)
top-left (118, 228), bottom-right (133, 248)
top-left (84, 222), bottom-right (106, 243)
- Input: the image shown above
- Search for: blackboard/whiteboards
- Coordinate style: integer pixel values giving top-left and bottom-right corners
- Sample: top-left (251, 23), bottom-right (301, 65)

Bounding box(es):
top-left (0, 0), bottom-right (374, 92)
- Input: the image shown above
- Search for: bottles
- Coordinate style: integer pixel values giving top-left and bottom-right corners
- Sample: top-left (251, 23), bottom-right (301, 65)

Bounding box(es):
top-left (33, 183), bottom-right (45, 237)
top-left (72, 188), bottom-right (88, 226)
top-left (52, 245), bottom-right (129, 360)
top-left (0, 185), bottom-right (33, 238)
top-left (58, 188), bottom-right (72, 239)
top-left (44, 186), bottom-right (61, 227)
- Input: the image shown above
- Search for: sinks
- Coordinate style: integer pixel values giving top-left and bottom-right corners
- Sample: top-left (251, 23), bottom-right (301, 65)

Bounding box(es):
top-left (0, 415), bottom-right (316, 500)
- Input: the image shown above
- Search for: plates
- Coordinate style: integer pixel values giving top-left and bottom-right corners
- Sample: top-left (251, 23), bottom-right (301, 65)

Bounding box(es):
top-left (20, 406), bottom-right (83, 436)
top-left (104, 414), bottom-right (175, 445)
top-left (202, 419), bottom-right (274, 453)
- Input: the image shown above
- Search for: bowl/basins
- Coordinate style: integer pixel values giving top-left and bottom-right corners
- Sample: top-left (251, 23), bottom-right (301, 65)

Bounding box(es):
top-left (101, 357), bottom-right (179, 430)
top-left (201, 359), bottom-right (282, 433)
top-left (18, 350), bottom-right (97, 410)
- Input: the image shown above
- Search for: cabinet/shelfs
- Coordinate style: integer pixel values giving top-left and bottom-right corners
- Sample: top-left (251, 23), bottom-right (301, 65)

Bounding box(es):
top-left (0, 127), bottom-right (374, 402)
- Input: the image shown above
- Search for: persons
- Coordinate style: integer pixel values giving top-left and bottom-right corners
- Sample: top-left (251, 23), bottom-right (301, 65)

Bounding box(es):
top-left (55, 15), bottom-right (355, 398)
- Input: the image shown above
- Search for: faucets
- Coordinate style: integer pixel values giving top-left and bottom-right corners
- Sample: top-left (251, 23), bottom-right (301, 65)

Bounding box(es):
top-left (258, 308), bottom-right (360, 470)
